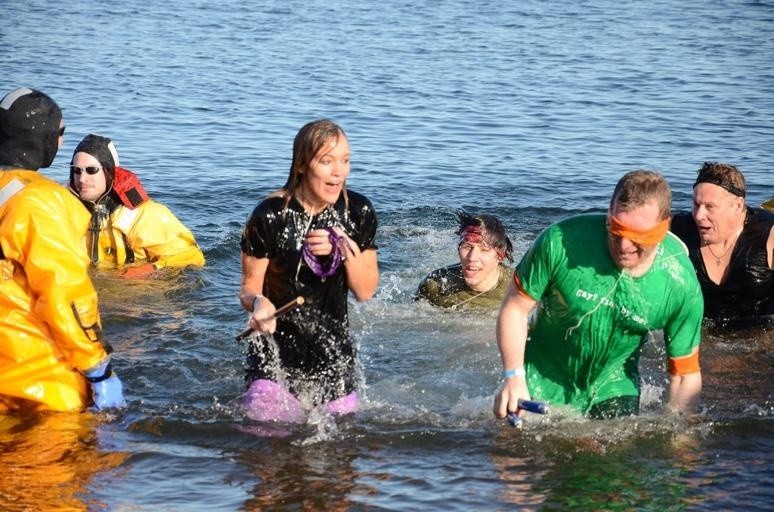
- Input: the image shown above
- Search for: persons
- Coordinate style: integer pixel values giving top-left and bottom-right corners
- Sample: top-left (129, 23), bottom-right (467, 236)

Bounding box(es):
top-left (58, 132), bottom-right (205, 279)
top-left (236, 117), bottom-right (382, 433)
top-left (664, 161), bottom-right (773, 339)
top-left (490, 168), bottom-right (704, 428)
top-left (1, 84), bottom-right (129, 423)
top-left (413, 205), bottom-right (524, 322)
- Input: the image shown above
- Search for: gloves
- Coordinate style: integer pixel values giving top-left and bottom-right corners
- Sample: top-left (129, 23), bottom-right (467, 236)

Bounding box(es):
top-left (85, 361), bottom-right (127, 412)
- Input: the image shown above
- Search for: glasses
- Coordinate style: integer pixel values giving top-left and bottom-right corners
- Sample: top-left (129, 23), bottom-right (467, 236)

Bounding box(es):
top-left (56, 126), bottom-right (65, 136)
top-left (70, 165), bottom-right (102, 175)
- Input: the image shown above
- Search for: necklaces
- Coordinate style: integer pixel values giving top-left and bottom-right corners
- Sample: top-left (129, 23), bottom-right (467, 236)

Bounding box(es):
top-left (706, 241), bottom-right (733, 266)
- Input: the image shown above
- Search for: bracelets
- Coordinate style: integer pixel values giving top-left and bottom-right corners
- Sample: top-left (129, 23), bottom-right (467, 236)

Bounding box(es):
top-left (502, 367), bottom-right (526, 378)
top-left (251, 294), bottom-right (265, 312)
